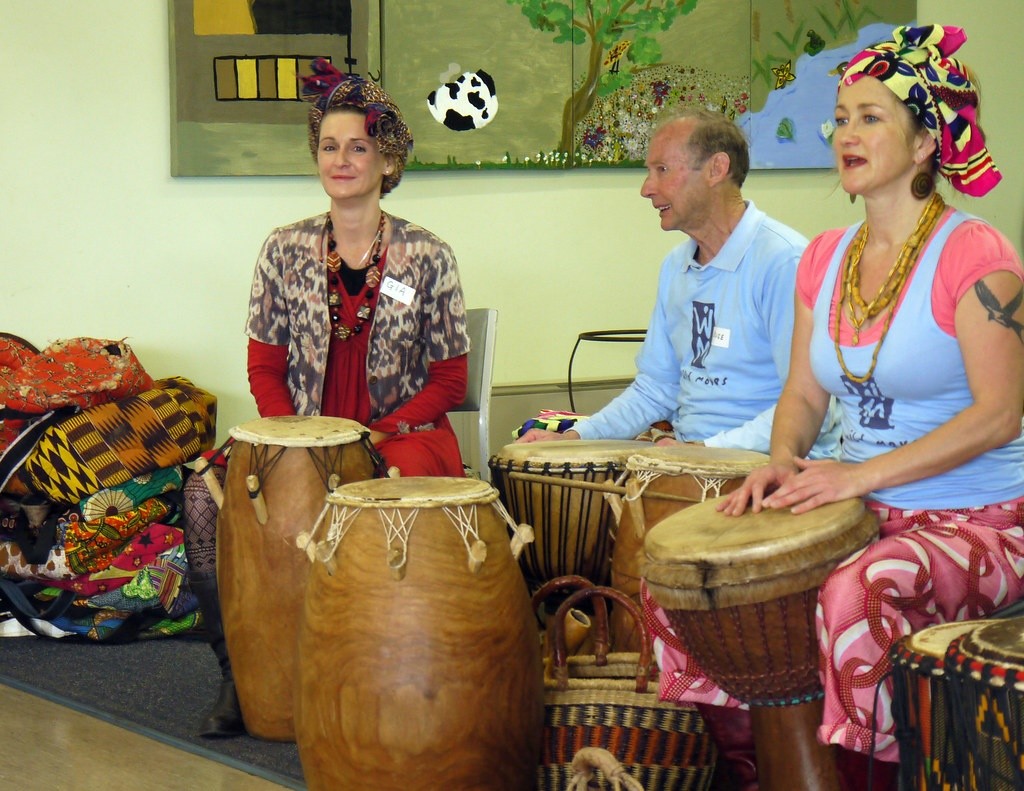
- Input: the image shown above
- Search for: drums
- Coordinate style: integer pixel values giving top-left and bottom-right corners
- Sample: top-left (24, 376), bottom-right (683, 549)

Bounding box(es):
top-left (293, 480), bottom-right (540, 789)
top-left (488, 439), bottom-right (670, 601)
top-left (892, 619), bottom-right (1001, 791)
top-left (942, 617), bottom-right (1023, 790)
top-left (615, 430), bottom-right (772, 646)
top-left (215, 416), bottom-right (384, 744)
top-left (638, 492), bottom-right (883, 791)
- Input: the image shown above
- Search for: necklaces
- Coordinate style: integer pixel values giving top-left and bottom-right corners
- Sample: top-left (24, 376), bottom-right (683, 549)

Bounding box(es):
top-left (325, 214), bottom-right (385, 341)
top-left (829, 195), bottom-right (944, 382)
top-left (357, 231), bottom-right (379, 266)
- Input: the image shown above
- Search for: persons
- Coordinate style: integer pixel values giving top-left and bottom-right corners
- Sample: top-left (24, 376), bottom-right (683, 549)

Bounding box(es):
top-left (640, 24), bottom-right (1024, 791)
top-left (514, 107), bottom-right (837, 455)
top-left (181, 72), bottom-right (471, 737)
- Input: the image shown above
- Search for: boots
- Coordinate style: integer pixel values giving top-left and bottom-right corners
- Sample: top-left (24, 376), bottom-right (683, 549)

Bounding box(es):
top-left (189, 569), bottom-right (245, 737)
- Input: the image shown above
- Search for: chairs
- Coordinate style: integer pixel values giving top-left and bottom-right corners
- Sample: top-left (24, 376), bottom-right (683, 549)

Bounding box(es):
top-left (446, 309), bottom-right (498, 485)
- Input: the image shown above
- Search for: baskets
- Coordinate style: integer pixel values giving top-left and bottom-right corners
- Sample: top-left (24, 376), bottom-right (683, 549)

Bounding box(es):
top-left (509, 575), bottom-right (719, 791)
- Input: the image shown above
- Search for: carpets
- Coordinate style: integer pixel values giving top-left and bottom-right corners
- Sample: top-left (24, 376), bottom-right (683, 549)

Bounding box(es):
top-left (0, 636), bottom-right (307, 791)
